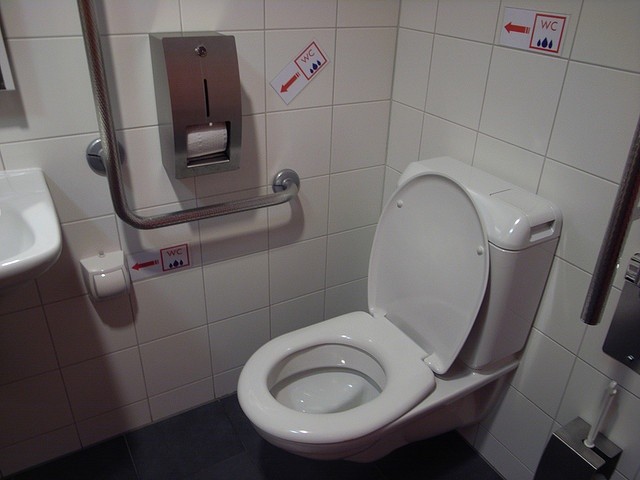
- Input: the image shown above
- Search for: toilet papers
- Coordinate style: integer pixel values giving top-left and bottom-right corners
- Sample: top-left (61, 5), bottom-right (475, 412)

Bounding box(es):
top-left (187, 122), bottom-right (229, 158)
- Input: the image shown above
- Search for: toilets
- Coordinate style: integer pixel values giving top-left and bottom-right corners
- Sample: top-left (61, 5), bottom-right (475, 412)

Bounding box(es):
top-left (236, 157), bottom-right (564, 463)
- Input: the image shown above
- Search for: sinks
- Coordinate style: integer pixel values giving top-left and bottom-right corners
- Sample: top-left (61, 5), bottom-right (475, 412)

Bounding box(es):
top-left (0, 165), bottom-right (63, 288)
